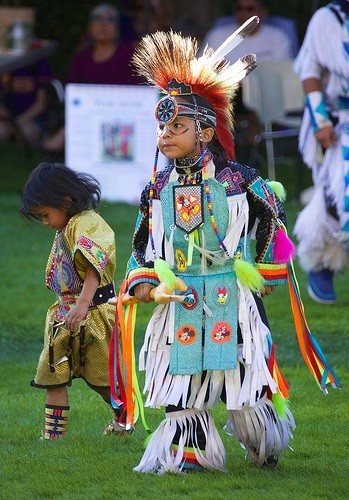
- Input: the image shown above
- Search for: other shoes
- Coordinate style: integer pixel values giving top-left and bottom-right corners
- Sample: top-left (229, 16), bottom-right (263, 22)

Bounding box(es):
top-left (306, 268), bottom-right (336, 304)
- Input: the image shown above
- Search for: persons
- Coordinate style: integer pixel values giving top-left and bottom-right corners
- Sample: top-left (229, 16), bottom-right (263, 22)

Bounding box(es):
top-left (42, 4), bottom-right (145, 161)
top-left (198, 0), bottom-right (292, 163)
top-left (0, 20), bottom-right (50, 160)
top-left (124, 15), bottom-right (297, 474)
top-left (18, 162), bottom-right (136, 441)
top-left (292, 0), bottom-right (349, 305)
